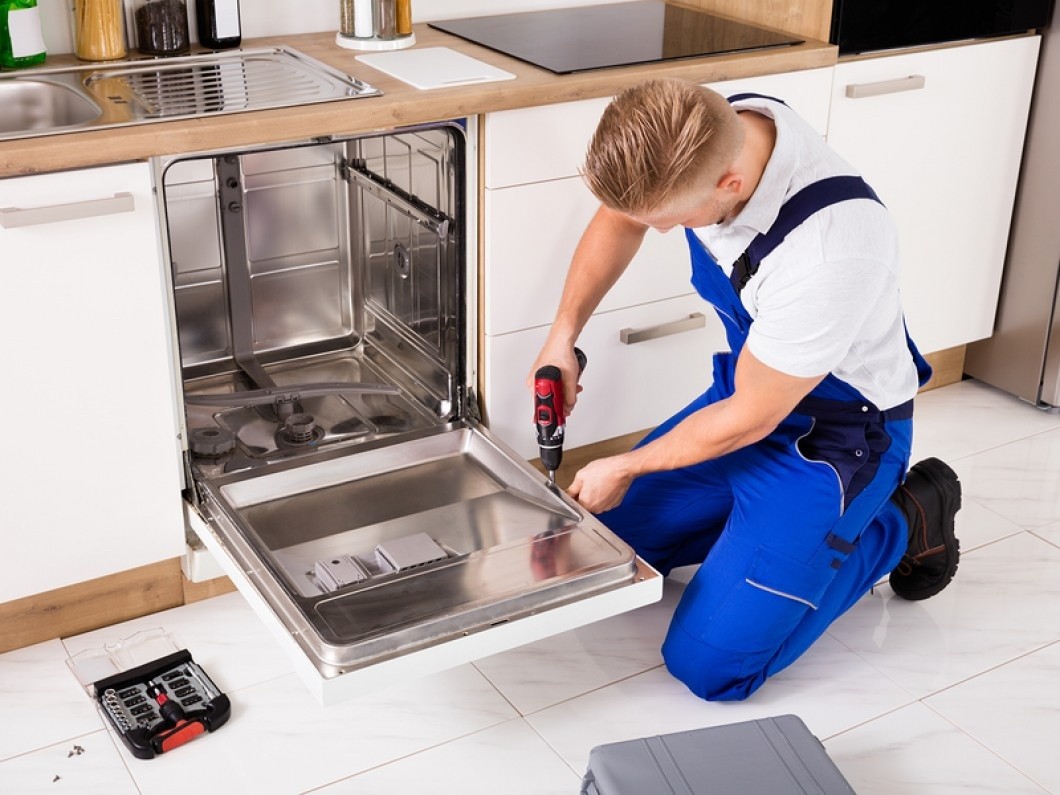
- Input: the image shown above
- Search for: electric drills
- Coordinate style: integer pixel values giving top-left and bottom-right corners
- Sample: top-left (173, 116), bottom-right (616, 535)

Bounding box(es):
top-left (536, 346), bottom-right (589, 492)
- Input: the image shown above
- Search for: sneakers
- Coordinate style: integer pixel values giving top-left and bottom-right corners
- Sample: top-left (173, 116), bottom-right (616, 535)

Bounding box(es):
top-left (890, 456), bottom-right (963, 600)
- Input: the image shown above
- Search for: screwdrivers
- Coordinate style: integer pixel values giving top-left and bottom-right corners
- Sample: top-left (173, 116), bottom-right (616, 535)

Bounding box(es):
top-left (148, 680), bottom-right (184, 725)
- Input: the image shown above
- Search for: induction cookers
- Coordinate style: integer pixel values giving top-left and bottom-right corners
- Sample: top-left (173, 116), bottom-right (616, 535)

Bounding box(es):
top-left (427, 0), bottom-right (807, 76)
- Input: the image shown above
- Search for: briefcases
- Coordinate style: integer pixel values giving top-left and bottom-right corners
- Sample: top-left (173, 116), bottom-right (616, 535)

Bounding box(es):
top-left (579, 714), bottom-right (856, 794)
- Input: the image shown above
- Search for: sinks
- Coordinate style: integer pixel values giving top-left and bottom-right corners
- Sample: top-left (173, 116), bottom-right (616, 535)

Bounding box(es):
top-left (2, 74), bottom-right (102, 142)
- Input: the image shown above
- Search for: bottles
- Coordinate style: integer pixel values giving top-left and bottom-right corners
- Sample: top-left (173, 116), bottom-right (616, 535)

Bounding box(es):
top-left (70, 0), bottom-right (129, 62)
top-left (134, 0), bottom-right (191, 55)
top-left (196, 0), bottom-right (243, 49)
top-left (0, 0), bottom-right (47, 69)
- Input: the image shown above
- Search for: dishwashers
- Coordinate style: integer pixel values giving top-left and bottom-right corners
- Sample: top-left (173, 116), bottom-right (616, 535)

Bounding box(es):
top-left (148, 114), bottom-right (664, 711)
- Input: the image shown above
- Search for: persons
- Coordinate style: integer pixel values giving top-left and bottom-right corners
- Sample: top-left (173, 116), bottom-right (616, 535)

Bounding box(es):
top-left (526, 74), bottom-right (962, 701)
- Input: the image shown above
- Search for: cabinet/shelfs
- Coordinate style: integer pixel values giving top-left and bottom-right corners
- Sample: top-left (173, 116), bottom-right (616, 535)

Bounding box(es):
top-left (475, 34), bottom-right (1048, 492)
top-left (0, 160), bottom-right (205, 657)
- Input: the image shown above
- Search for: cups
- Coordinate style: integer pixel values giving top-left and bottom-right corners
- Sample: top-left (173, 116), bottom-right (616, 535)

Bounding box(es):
top-left (338, 0), bottom-right (414, 41)
